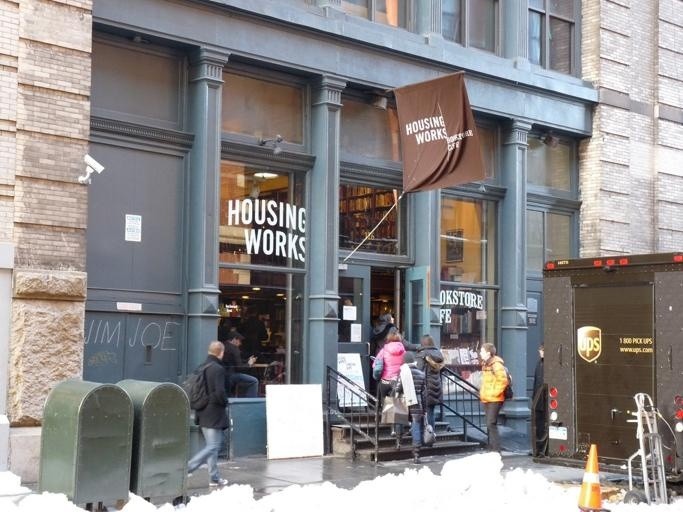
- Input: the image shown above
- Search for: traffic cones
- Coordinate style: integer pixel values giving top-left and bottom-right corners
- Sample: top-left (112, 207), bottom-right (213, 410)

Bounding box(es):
top-left (571, 442), bottom-right (612, 512)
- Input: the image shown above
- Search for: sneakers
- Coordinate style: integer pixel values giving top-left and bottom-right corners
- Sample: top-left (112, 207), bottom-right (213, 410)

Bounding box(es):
top-left (209, 477), bottom-right (229, 487)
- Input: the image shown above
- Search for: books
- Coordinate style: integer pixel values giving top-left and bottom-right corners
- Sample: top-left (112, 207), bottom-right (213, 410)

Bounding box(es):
top-left (439, 266), bottom-right (481, 392)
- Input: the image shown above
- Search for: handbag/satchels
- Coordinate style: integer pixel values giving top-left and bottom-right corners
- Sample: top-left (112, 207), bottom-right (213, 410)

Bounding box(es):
top-left (372, 358), bottom-right (383, 381)
top-left (422, 414), bottom-right (436, 448)
top-left (380, 393), bottom-right (409, 426)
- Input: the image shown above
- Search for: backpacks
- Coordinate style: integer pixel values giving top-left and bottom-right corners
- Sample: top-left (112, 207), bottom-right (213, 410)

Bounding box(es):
top-left (490, 363), bottom-right (513, 399)
top-left (183, 363), bottom-right (216, 411)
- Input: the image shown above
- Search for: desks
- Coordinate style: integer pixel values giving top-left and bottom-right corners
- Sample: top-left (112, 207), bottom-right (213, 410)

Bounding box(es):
top-left (252, 364), bottom-right (270, 396)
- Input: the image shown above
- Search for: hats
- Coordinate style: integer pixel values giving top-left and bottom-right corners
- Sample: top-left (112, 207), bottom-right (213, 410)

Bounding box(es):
top-left (229, 332), bottom-right (245, 341)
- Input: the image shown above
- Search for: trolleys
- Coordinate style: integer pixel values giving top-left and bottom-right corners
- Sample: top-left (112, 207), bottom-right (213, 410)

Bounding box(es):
top-left (620, 389), bottom-right (674, 505)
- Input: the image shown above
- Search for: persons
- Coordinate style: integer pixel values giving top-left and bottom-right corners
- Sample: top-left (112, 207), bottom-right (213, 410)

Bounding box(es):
top-left (241, 307), bottom-right (267, 352)
top-left (369, 312), bottom-right (444, 464)
top-left (220, 332), bottom-right (258, 398)
top-left (526, 343), bottom-right (547, 456)
top-left (187, 340), bottom-right (229, 486)
top-left (479, 343), bottom-right (508, 453)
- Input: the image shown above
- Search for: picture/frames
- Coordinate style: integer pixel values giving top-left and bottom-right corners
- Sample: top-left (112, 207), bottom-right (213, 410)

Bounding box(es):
top-left (445, 229), bottom-right (464, 262)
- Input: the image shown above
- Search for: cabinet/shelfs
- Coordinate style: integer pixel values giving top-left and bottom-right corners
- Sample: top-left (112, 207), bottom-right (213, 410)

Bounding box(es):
top-left (338, 182), bottom-right (397, 254)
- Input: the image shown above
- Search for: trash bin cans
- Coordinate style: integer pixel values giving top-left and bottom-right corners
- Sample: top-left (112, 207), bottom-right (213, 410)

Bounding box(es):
top-left (37, 379), bottom-right (191, 512)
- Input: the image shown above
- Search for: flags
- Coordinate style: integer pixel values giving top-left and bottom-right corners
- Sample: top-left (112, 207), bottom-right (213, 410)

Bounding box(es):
top-left (392, 71), bottom-right (485, 192)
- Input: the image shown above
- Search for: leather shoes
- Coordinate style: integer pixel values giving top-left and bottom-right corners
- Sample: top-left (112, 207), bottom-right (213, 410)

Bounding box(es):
top-left (392, 430), bottom-right (421, 463)
